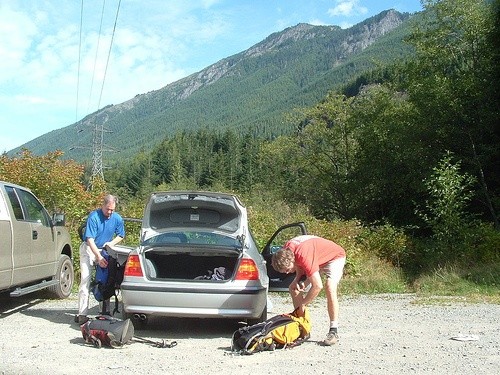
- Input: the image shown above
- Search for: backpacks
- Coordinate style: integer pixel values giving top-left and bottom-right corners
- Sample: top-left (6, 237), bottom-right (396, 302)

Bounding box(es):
top-left (93, 246), bottom-right (121, 303)
top-left (231, 306), bottom-right (312, 355)
top-left (80, 316), bottom-right (134, 349)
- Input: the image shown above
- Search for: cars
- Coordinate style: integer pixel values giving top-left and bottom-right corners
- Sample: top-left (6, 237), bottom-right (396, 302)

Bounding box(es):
top-left (78, 190), bottom-right (309, 332)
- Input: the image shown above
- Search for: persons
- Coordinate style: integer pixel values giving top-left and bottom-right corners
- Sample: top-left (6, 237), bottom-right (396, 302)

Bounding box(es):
top-left (73, 195), bottom-right (125, 324)
top-left (271, 235), bottom-right (346, 346)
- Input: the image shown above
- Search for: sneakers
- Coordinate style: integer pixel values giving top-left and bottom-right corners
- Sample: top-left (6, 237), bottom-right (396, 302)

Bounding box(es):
top-left (324, 331), bottom-right (340, 345)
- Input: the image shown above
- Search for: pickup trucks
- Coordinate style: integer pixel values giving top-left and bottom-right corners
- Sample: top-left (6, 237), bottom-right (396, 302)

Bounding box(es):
top-left (0, 180), bottom-right (75, 300)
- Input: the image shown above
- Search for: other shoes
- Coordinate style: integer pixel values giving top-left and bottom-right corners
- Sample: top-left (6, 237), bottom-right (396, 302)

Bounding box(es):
top-left (79, 315), bottom-right (86, 325)
top-left (98, 311), bottom-right (113, 317)
